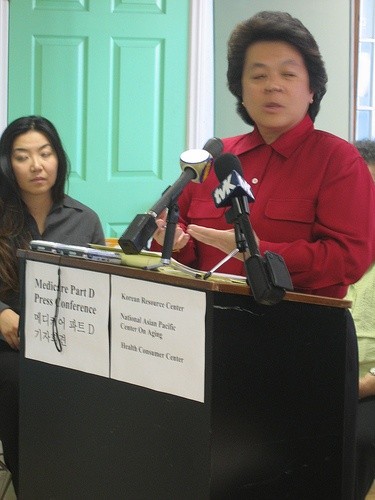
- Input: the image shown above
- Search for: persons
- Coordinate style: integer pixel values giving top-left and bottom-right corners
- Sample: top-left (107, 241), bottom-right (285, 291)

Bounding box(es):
top-left (0, 116), bottom-right (106, 500)
top-left (341, 140), bottom-right (375, 500)
top-left (149, 10), bottom-right (375, 300)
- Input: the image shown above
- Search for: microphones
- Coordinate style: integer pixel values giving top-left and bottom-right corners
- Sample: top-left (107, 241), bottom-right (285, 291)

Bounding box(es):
top-left (214, 154), bottom-right (293, 306)
top-left (119, 137), bottom-right (225, 255)
